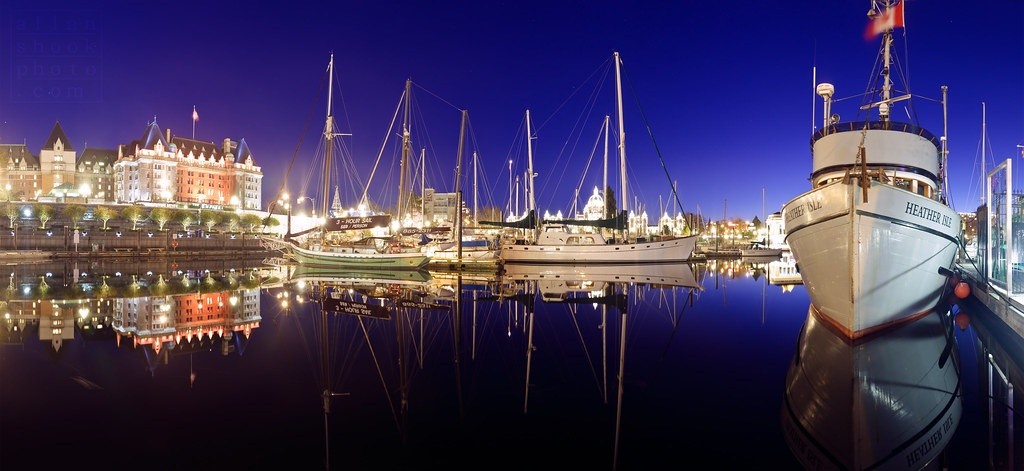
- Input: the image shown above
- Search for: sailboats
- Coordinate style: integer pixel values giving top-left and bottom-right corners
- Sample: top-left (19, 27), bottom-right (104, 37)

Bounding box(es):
top-left (270, 273), bottom-right (698, 454)
top-left (261, 52), bottom-right (706, 302)
top-left (733, 186), bottom-right (787, 259)
top-left (702, 200), bottom-right (748, 258)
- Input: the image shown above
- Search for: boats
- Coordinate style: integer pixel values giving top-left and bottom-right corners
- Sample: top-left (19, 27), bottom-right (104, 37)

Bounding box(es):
top-left (780, 1), bottom-right (970, 345)
top-left (786, 300), bottom-right (965, 471)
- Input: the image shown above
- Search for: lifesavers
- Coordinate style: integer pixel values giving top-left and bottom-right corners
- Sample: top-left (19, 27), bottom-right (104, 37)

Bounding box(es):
top-left (389, 241), bottom-right (401, 254)
top-left (389, 284), bottom-right (400, 295)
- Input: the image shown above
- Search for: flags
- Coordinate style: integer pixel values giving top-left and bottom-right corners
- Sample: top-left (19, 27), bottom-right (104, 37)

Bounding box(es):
top-left (192, 110), bottom-right (199, 122)
top-left (864, 0), bottom-right (903, 42)
